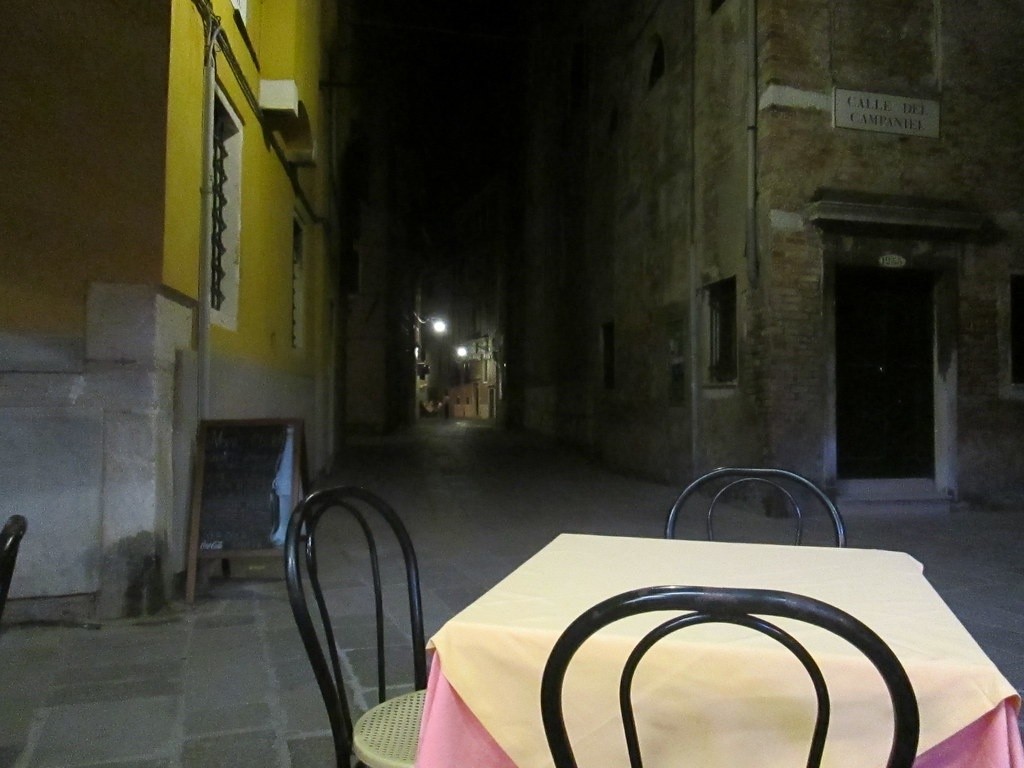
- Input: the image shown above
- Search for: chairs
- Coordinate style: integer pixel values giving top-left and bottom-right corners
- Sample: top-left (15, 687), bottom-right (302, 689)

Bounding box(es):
top-left (539, 585), bottom-right (920, 768)
top-left (665, 467), bottom-right (849, 548)
top-left (282, 483), bottom-right (429, 768)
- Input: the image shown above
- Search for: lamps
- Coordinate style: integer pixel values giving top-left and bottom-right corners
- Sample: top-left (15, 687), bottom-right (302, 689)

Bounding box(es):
top-left (414, 311), bottom-right (447, 335)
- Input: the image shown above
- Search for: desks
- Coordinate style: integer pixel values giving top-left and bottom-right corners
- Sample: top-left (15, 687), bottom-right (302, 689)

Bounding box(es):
top-left (413, 532), bottom-right (1024, 768)
